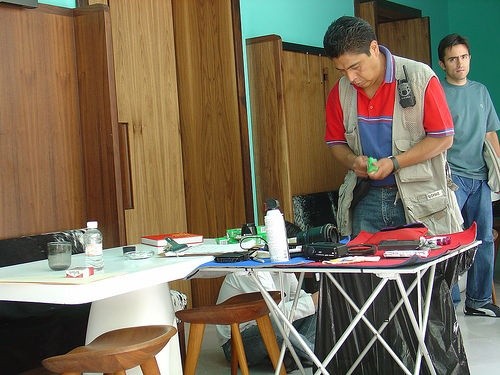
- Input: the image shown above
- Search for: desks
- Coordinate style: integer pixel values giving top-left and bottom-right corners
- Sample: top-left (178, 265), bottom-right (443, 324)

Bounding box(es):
top-left (199, 240), bottom-right (483, 375)
top-left (0, 239), bottom-right (256, 375)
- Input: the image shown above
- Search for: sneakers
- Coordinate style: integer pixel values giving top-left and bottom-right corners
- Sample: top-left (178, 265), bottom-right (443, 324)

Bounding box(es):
top-left (463, 303), bottom-right (500, 318)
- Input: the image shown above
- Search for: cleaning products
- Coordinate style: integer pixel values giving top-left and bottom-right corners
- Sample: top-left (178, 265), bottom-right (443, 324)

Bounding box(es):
top-left (262, 196), bottom-right (289, 263)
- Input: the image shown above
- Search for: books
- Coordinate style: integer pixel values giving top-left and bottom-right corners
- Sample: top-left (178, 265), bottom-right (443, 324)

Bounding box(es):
top-left (141, 233), bottom-right (203, 247)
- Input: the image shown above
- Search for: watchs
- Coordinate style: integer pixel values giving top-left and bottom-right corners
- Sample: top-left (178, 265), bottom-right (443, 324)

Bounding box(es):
top-left (388, 156), bottom-right (401, 174)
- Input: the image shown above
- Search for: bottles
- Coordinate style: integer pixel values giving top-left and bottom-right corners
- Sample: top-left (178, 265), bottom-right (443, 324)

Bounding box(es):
top-left (263, 200), bottom-right (290, 263)
top-left (83, 221), bottom-right (104, 271)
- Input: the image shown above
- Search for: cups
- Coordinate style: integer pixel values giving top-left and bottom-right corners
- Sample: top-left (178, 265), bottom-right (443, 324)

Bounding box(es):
top-left (47, 242), bottom-right (72, 271)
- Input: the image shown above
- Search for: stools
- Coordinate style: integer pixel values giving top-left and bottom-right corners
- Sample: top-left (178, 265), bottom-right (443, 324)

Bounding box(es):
top-left (42, 325), bottom-right (177, 375)
top-left (175, 292), bottom-right (287, 375)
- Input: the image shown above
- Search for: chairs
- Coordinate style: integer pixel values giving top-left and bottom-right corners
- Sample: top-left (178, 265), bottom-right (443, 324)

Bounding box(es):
top-left (292, 190), bottom-right (340, 232)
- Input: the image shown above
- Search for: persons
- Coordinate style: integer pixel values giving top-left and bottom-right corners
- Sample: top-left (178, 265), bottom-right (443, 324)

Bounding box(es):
top-left (323, 16), bottom-right (464, 241)
top-left (437, 33), bottom-right (500, 317)
top-left (215, 221), bottom-right (319, 375)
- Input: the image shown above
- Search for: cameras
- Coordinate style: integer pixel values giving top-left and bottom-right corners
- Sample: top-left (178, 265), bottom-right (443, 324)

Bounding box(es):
top-left (302, 241), bottom-right (348, 261)
